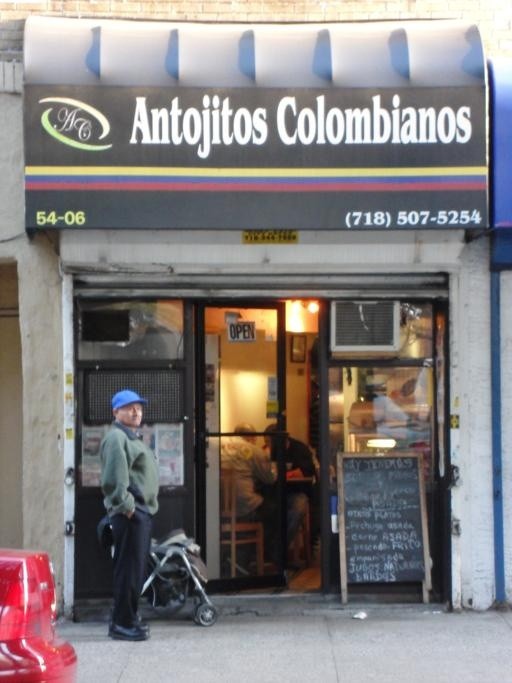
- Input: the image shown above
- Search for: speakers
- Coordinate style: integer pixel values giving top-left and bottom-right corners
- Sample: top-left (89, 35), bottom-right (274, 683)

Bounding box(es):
top-left (81, 309), bottom-right (130, 342)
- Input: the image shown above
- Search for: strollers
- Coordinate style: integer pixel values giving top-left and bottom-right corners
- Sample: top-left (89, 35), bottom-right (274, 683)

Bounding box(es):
top-left (98, 514), bottom-right (219, 627)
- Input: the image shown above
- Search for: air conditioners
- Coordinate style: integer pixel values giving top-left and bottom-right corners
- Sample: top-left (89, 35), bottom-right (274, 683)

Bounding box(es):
top-left (329, 300), bottom-right (400, 360)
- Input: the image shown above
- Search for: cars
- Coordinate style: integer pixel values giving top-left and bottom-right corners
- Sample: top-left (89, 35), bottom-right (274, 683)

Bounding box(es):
top-left (0, 547), bottom-right (78, 683)
top-left (348, 402), bottom-right (431, 453)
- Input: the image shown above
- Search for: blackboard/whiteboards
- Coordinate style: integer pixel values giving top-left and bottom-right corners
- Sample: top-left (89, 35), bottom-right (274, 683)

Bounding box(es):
top-left (337, 452), bottom-right (431, 584)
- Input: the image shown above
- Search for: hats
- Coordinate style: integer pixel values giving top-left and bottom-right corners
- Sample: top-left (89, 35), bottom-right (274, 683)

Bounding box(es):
top-left (111, 389), bottom-right (147, 410)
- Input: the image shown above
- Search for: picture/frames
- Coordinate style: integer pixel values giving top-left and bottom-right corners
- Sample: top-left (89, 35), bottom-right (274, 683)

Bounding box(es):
top-left (290, 335), bottom-right (307, 363)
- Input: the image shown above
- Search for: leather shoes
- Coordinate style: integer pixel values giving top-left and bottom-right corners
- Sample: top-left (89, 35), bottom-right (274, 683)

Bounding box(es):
top-left (109, 619), bottom-right (150, 641)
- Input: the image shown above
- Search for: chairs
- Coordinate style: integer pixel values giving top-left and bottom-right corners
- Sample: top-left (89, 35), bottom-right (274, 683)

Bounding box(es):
top-left (220, 476), bottom-right (264, 578)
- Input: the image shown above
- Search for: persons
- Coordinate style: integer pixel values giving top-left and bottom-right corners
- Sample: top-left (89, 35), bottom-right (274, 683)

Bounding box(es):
top-left (98, 388), bottom-right (160, 642)
top-left (262, 424), bottom-right (322, 573)
top-left (219, 421), bottom-right (292, 583)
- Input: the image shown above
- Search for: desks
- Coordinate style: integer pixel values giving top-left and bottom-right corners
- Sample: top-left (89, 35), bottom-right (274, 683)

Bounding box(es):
top-left (263, 476), bottom-right (318, 569)
